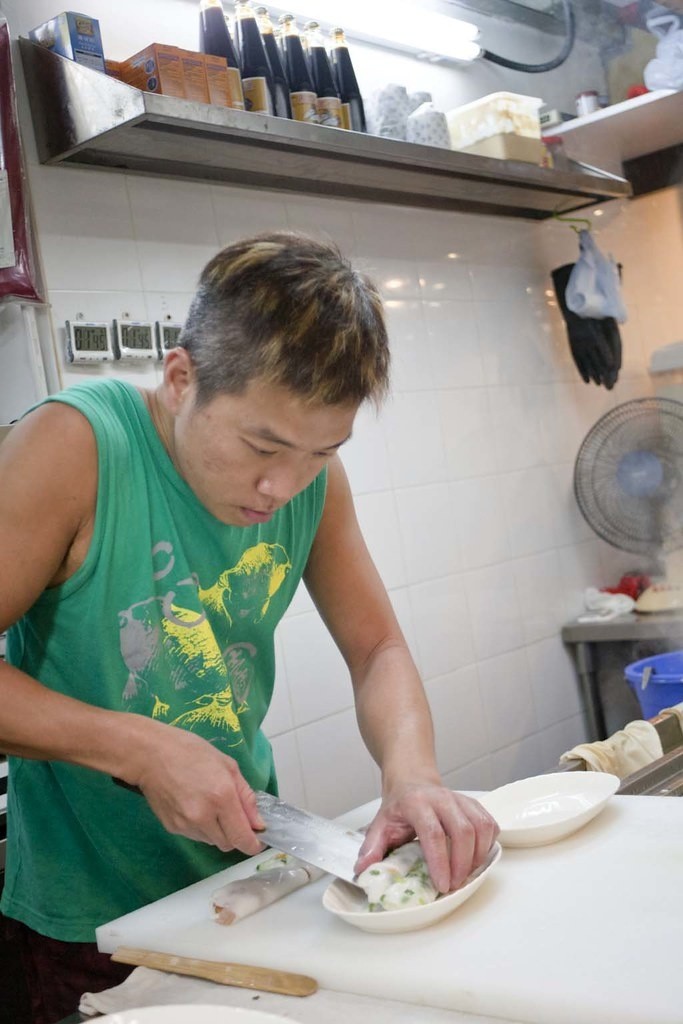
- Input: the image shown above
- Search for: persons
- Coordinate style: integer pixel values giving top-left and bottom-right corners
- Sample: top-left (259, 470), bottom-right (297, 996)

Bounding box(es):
top-left (0, 233), bottom-right (500, 1024)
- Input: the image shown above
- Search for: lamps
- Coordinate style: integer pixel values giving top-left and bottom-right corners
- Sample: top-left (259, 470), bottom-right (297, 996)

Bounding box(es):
top-left (253, 0), bottom-right (487, 65)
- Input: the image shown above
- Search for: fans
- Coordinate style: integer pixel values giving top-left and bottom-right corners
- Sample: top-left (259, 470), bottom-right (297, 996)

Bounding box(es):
top-left (572, 396), bottom-right (683, 615)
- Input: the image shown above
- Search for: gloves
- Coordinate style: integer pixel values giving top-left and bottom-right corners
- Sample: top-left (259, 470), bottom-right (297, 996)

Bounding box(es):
top-left (551, 263), bottom-right (623, 390)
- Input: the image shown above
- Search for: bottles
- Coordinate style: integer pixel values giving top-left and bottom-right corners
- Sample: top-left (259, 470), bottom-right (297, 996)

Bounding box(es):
top-left (198, 0), bottom-right (367, 134)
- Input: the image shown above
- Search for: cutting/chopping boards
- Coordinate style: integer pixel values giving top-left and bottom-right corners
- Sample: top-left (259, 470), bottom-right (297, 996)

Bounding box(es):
top-left (95, 787), bottom-right (683, 1024)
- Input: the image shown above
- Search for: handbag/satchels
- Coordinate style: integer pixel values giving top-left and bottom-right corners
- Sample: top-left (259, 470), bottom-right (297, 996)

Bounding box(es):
top-left (643, 15), bottom-right (683, 91)
top-left (564, 228), bottom-right (627, 324)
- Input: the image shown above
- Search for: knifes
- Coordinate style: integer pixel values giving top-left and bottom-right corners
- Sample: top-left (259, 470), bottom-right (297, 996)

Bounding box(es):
top-left (111, 776), bottom-right (368, 889)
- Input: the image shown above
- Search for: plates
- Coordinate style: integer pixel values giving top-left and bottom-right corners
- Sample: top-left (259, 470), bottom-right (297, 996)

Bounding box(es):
top-left (322, 840), bottom-right (502, 933)
top-left (478, 770), bottom-right (620, 848)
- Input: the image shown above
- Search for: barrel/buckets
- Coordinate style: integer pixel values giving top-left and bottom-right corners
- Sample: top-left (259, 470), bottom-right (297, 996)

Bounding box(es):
top-left (624, 650), bottom-right (683, 720)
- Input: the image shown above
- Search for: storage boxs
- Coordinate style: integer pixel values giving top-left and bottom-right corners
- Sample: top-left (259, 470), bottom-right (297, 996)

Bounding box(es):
top-left (25, 10), bottom-right (231, 107)
top-left (448, 91), bottom-right (547, 166)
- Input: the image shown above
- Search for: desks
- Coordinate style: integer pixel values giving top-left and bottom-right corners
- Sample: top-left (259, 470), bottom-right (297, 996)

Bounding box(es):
top-left (562, 617), bottom-right (683, 744)
